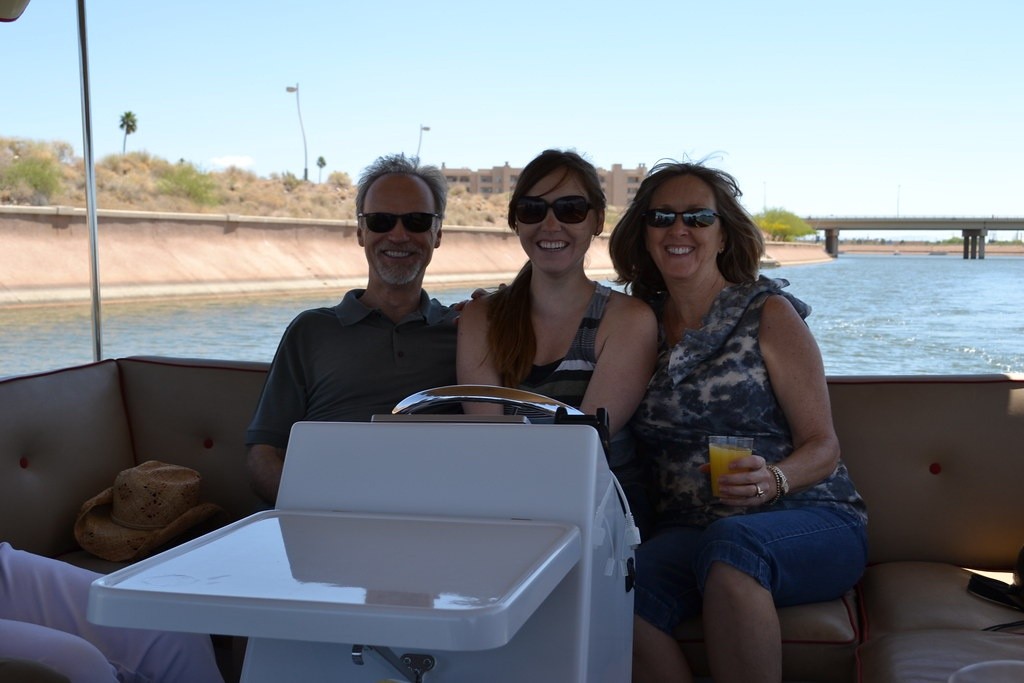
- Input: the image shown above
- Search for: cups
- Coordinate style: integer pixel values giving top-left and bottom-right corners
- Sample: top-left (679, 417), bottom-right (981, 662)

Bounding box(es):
top-left (708, 435), bottom-right (754, 497)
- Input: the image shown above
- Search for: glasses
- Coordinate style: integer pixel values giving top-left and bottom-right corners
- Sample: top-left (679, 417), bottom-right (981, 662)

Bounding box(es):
top-left (358, 212), bottom-right (439, 233)
top-left (515, 196), bottom-right (595, 224)
top-left (642, 208), bottom-right (720, 228)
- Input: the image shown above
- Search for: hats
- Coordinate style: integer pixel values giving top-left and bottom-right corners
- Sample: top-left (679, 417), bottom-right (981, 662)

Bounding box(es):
top-left (74, 458), bottom-right (230, 563)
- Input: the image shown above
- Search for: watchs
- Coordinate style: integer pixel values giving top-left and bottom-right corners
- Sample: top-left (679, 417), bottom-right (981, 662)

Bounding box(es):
top-left (772, 463), bottom-right (789, 501)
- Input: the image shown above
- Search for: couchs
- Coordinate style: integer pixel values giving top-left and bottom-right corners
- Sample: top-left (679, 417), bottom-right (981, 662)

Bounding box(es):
top-left (0, 354), bottom-right (1024, 683)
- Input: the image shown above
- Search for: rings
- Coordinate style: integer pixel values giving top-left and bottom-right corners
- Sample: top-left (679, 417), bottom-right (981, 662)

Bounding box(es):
top-left (755, 483), bottom-right (764, 498)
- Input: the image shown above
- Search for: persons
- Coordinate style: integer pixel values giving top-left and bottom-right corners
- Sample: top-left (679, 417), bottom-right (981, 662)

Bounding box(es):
top-left (0, 542), bottom-right (224, 683)
top-left (241, 154), bottom-right (506, 506)
top-left (451, 164), bottom-right (872, 683)
top-left (456, 150), bottom-right (658, 478)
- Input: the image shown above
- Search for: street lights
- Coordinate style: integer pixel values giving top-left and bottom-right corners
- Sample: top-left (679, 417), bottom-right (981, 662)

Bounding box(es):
top-left (417, 124), bottom-right (431, 156)
top-left (286, 82), bottom-right (308, 180)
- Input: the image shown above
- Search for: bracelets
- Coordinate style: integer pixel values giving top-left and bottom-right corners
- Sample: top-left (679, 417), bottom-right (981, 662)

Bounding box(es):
top-left (766, 466), bottom-right (781, 506)
